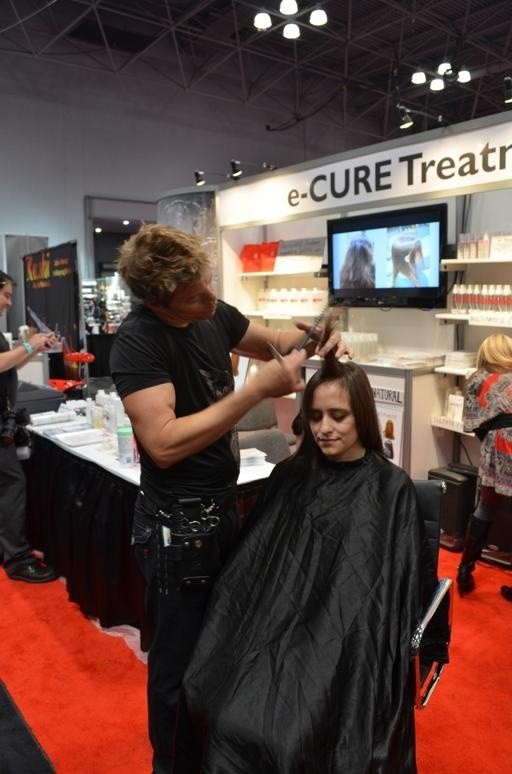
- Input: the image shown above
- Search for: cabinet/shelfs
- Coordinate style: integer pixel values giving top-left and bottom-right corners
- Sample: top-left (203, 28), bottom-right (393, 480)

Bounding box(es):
top-left (296, 353), bottom-right (462, 480)
top-left (237, 268), bottom-right (328, 320)
top-left (431, 259), bottom-right (512, 438)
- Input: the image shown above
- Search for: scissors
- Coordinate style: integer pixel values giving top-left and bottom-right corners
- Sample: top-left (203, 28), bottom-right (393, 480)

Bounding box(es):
top-left (267, 341), bottom-right (285, 364)
top-left (154, 498), bottom-right (220, 534)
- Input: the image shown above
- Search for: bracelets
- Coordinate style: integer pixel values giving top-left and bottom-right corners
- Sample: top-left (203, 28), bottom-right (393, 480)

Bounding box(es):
top-left (23, 343), bottom-right (32, 354)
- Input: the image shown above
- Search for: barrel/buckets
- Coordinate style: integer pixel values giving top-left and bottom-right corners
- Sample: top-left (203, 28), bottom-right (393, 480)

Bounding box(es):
top-left (85, 389), bottom-right (125, 433)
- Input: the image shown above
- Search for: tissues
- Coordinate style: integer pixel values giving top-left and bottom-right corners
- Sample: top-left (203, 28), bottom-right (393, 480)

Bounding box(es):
top-left (339, 332), bottom-right (379, 360)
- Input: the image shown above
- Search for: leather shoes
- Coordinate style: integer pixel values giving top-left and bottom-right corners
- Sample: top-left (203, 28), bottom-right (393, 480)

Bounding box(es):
top-left (10, 561), bottom-right (57, 582)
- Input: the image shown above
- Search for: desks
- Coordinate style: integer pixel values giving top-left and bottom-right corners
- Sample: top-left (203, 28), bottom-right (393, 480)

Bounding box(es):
top-left (84, 333), bottom-right (118, 378)
top-left (14, 412), bottom-right (296, 652)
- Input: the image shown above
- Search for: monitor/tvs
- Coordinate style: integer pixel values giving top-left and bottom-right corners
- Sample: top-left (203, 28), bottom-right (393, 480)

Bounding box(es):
top-left (327, 203), bottom-right (448, 309)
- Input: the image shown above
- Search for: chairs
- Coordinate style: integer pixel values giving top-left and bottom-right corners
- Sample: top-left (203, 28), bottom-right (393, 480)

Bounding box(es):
top-left (236, 397), bottom-right (294, 468)
top-left (406, 476), bottom-right (453, 710)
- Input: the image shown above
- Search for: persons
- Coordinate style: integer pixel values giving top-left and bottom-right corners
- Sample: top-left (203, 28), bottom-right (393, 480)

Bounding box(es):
top-left (383, 419), bottom-right (395, 459)
top-left (109, 224), bottom-right (358, 773)
top-left (340, 240), bottom-right (375, 288)
top-left (456, 333), bottom-right (512, 602)
top-left (391, 236), bottom-right (429, 288)
top-left (283, 316), bottom-right (382, 469)
top-left (0, 271), bottom-right (58, 584)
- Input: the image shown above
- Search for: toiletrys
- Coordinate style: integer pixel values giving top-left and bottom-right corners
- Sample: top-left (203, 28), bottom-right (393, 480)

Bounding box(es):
top-left (443, 385), bottom-right (466, 423)
top-left (256, 287), bottom-right (328, 314)
top-left (456, 232), bottom-right (490, 260)
top-left (449, 282), bottom-right (512, 317)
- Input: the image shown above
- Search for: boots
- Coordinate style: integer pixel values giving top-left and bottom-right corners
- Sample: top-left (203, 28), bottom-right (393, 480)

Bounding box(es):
top-left (457, 515), bottom-right (490, 598)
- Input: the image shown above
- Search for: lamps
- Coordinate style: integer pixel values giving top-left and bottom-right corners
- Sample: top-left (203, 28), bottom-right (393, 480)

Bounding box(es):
top-left (192, 168), bottom-right (231, 186)
top-left (396, 102), bottom-right (449, 133)
top-left (502, 74), bottom-right (512, 104)
top-left (228, 157), bottom-right (279, 180)
